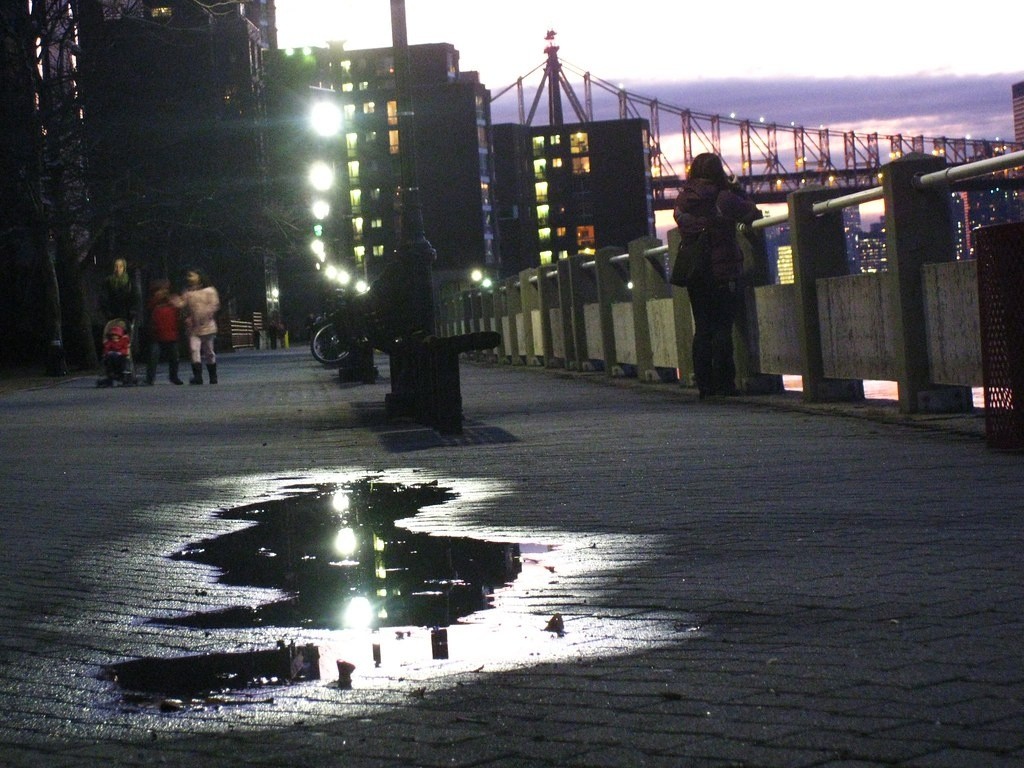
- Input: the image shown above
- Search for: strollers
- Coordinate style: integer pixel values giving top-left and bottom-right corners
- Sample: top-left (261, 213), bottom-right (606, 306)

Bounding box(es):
top-left (96, 314), bottom-right (137, 389)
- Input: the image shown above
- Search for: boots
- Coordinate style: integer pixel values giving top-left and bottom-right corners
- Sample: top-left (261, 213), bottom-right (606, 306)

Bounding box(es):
top-left (190, 363), bottom-right (203, 384)
top-left (169, 357), bottom-right (183, 385)
top-left (144, 360), bottom-right (156, 384)
top-left (206, 363), bottom-right (217, 383)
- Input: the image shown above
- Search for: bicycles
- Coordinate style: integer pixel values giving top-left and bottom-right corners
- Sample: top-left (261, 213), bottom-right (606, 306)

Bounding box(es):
top-left (310, 291), bottom-right (351, 366)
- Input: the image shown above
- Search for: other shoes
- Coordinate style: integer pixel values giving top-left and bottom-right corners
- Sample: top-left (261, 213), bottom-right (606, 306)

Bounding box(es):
top-left (122, 370), bottom-right (132, 375)
top-left (698, 382), bottom-right (740, 399)
top-left (102, 375), bottom-right (108, 380)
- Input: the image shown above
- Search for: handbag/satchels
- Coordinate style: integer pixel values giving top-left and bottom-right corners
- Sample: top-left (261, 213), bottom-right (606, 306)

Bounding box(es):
top-left (669, 226), bottom-right (708, 288)
top-left (142, 318), bottom-right (158, 343)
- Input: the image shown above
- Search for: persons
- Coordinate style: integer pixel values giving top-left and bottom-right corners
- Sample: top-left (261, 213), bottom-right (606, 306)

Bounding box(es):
top-left (673, 153), bottom-right (763, 397)
top-left (252, 327), bottom-right (261, 350)
top-left (268, 320), bottom-right (286, 349)
top-left (145, 278), bottom-right (183, 386)
top-left (305, 314), bottom-right (316, 344)
top-left (169, 269), bottom-right (220, 385)
top-left (98, 254), bottom-right (140, 387)
